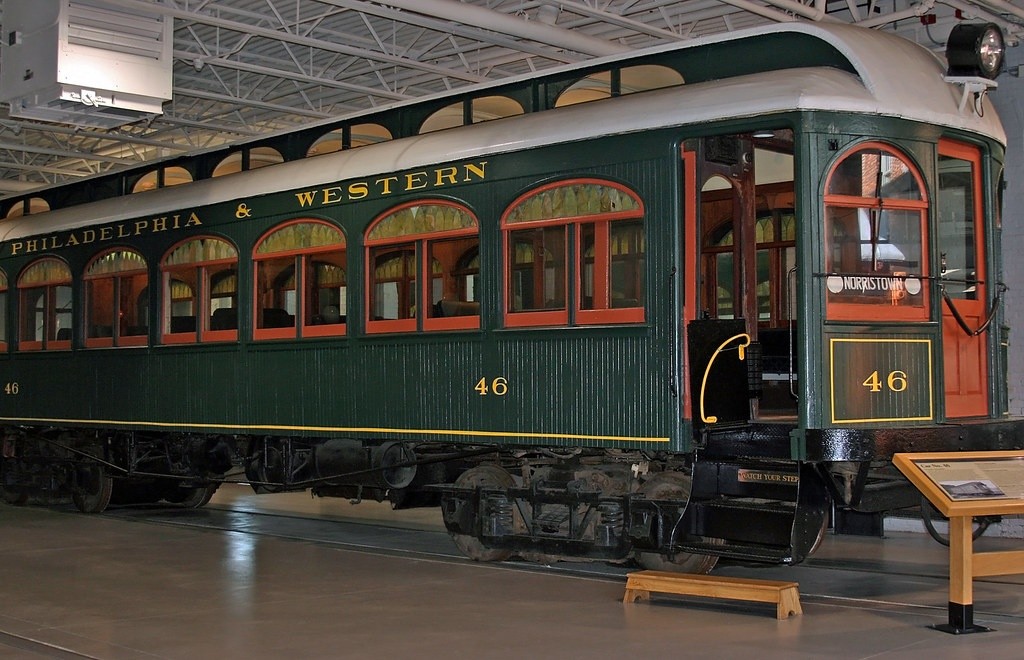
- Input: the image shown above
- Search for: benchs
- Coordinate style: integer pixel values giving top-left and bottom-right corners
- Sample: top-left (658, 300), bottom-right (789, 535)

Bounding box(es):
top-left (623, 570), bottom-right (803, 620)
top-left (55, 296), bottom-right (632, 340)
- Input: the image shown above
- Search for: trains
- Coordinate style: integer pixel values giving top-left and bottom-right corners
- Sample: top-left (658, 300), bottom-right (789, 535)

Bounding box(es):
top-left (0, 21), bottom-right (1016, 575)
